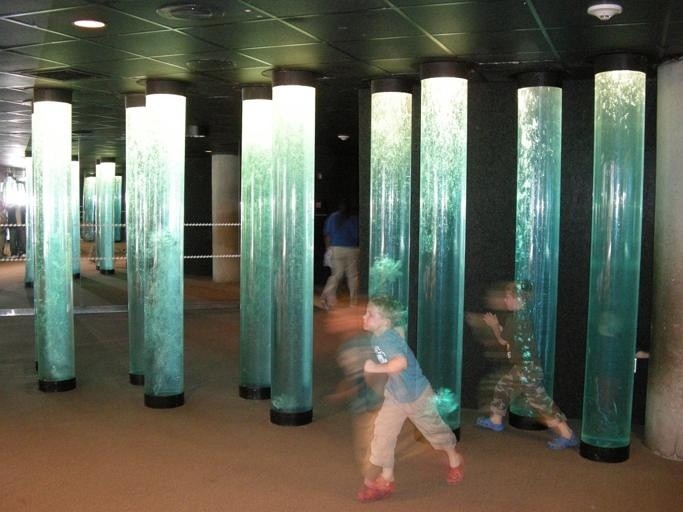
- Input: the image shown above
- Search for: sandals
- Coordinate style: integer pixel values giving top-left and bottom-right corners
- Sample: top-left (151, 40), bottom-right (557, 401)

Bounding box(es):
top-left (547, 431), bottom-right (581, 450)
top-left (477, 417), bottom-right (504, 431)
top-left (359, 478), bottom-right (391, 501)
top-left (447, 455), bottom-right (464, 484)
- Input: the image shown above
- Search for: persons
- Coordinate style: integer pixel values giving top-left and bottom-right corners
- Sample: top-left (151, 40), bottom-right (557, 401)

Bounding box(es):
top-left (318, 193), bottom-right (361, 311)
top-left (355, 295), bottom-right (465, 502)
top-left (0, 198), bottom-right (27, 260)
top-left (475, 280), bottom-right (578, 449)
